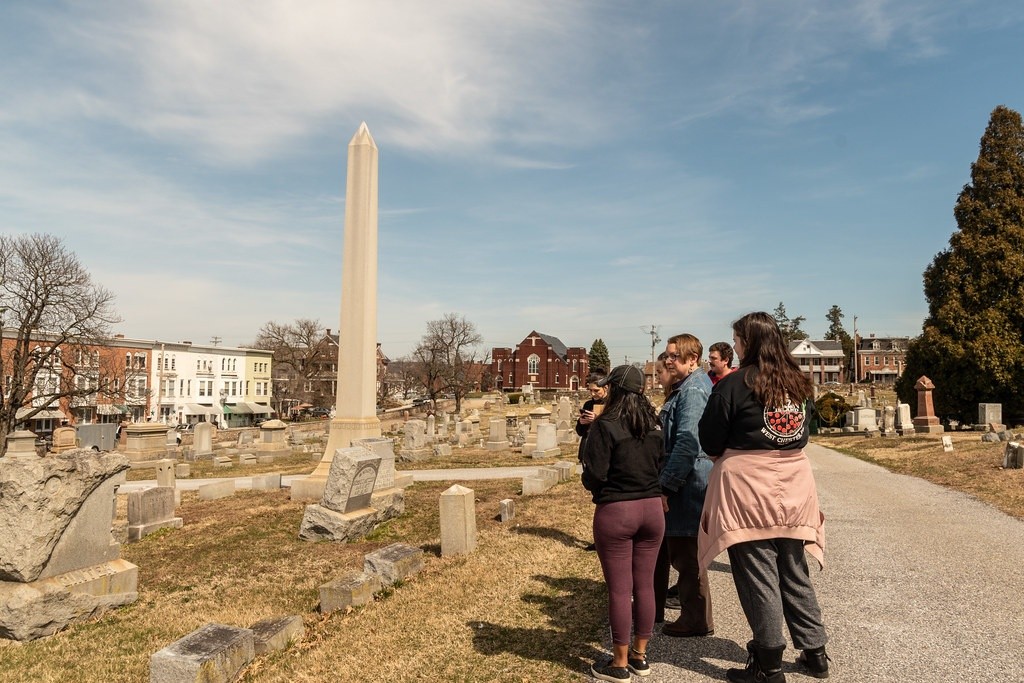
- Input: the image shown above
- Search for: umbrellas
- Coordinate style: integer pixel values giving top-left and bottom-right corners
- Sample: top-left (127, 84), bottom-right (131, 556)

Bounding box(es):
top-left (291, 403), bottom-right (313, 414)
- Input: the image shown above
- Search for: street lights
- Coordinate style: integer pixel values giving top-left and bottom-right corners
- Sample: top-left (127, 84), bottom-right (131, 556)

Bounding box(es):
top-left (651, 337), bottom-right (662, 389)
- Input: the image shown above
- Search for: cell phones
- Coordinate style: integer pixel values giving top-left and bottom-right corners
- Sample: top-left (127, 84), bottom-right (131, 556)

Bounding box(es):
top-left (580, 409), bottom-right (587, 419)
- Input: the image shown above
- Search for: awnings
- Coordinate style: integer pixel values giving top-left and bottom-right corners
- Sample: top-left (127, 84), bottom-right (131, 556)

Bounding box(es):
top-left (5, 407), bottom-right (66, 419)
top-left (97, 404), bottom-right (128, 414)
top-left (184, 402), bottom-right (275, 414)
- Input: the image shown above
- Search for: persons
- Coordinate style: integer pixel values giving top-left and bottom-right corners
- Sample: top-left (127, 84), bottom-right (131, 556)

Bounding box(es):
top-left (697, 311), bottom-right (831, 683)
top-left (116, 421), bottom-right (122, 434)
top-left (41, 436), bottom-right (50, 452)
top-left (576, 333), bottom-right (714, 683)
top-left (707, 342), bottom-right (738, 385)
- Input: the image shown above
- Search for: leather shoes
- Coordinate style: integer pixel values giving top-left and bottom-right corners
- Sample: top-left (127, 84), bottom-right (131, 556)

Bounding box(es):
top-left (663, 617), bottom-right (708, 637)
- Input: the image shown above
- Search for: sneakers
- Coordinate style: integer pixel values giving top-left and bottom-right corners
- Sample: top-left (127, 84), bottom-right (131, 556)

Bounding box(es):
top-left (627, 653), bottom-right (651, 676)
top-left (591, 659), bottom-right (631, 683)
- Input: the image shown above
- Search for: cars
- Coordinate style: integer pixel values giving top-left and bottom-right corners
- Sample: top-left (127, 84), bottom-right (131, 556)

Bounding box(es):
top-left (250, 418), bottom-right (272, 427)
top-left (310, 408), bottom-right (331, 417)
top-left (175, 423), bottom-right (195, 434)
top-left (376, 405), bottom-right (386, 414)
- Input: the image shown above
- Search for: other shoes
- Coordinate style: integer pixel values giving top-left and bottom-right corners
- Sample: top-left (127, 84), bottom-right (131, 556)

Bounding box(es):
top-left (665, 583), bottom-right (682, 610)
top-left (630, 602), bottom-right (664, 622)
top-left (708, 630), bottom-right (714, 635)
top-left (585, 543), bottom-right (596, 551)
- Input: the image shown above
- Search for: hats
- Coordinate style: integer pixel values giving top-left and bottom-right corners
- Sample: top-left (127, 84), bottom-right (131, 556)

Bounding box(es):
top-left (597, 365), bottom-right (645, 394)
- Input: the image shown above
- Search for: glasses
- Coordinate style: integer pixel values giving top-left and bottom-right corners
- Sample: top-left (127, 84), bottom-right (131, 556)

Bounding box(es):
top-left (662, 353), bottom-right (691, 361)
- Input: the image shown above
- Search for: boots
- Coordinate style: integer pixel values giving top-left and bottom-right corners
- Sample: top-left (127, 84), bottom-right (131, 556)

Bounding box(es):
top-left (802, 645), bottom-right (832, 678)
top-left (727, 641), bottom-right (786, 683)
